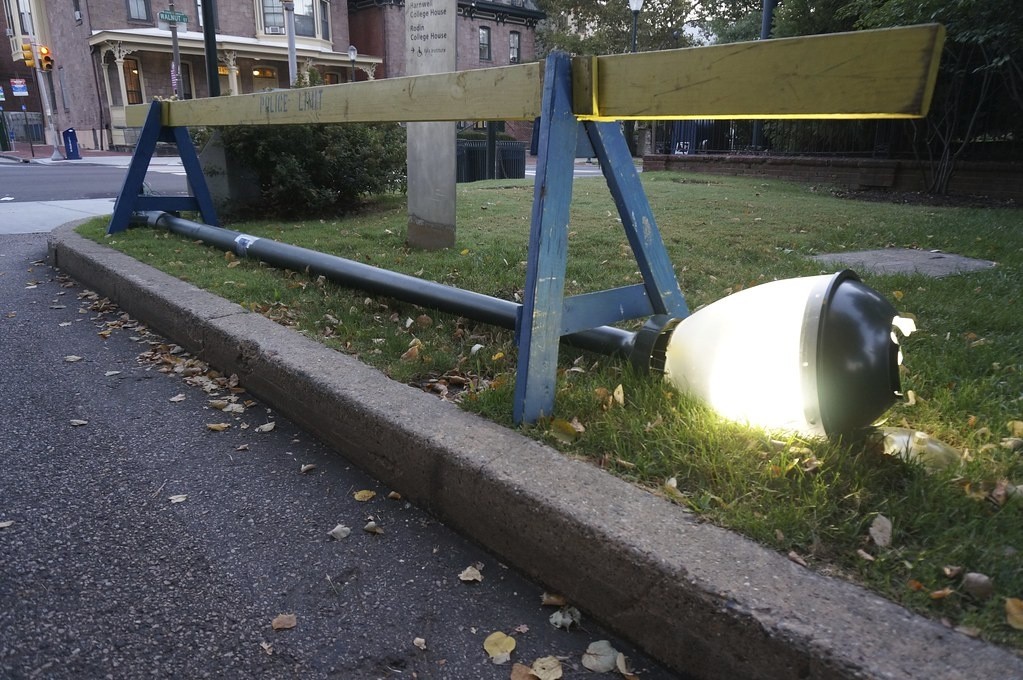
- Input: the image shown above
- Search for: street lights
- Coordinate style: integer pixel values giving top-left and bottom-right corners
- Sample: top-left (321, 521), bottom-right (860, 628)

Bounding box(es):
top-left (347, 45), bottom-right (358, 82)
top-left (622, 0), bottom-right (644, 146)
top-left (109, 179), bottom-right (917, 447)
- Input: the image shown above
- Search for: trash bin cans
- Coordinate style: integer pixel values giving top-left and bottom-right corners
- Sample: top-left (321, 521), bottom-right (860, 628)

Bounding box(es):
top-left (62, 127), bottom-right (82, 160)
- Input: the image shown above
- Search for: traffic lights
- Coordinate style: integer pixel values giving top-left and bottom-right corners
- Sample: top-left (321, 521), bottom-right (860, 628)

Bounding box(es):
top-left (21, 43), bottom-right (35, 68)
top-left (39, 46), bottom-right (52, 71)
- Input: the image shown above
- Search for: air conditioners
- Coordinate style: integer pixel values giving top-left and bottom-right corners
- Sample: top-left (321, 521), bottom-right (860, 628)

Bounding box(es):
top-left (270, 27), bottom-right (280, 34)
